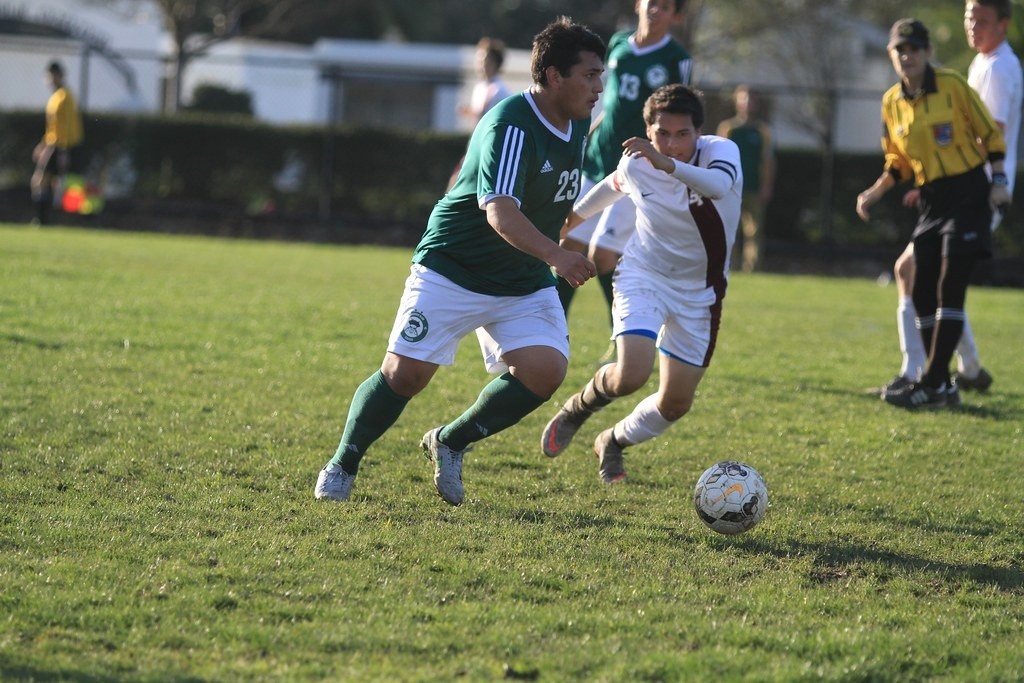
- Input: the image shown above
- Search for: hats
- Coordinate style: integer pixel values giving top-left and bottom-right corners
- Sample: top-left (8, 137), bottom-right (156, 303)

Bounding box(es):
top-left (887, 19), bottom-right (928, 50)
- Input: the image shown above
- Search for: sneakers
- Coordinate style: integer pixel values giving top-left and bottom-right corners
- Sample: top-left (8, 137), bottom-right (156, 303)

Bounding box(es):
top-left (541, 393), bottom-right (585, 459)
top-left (314, 459), bottom-right (356, 502)
top-left (419, 426), bottom-right (473, 506)
top-left (880, 381), bottom-right (947, 410)
top-left (864, 377), bottom-right (910, 399)
top-left (946, 383), bottom-right (960, 405)
top-left (949, 369), bottom-right (992, 393)
top-left (593, 428), bottom-right (625, 485)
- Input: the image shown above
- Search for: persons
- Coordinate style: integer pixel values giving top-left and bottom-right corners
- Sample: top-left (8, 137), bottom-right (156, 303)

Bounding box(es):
top-left (314, 0), bottom-right (776, 508)
top-left (854, 0), bottom-right (1024, 411)
top-left (31, 62), bottom-right (83, 228)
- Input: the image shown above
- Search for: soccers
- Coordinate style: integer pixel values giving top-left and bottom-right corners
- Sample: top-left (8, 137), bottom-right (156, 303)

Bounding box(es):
top-left (694, 460), bottom-right (768, 535)
